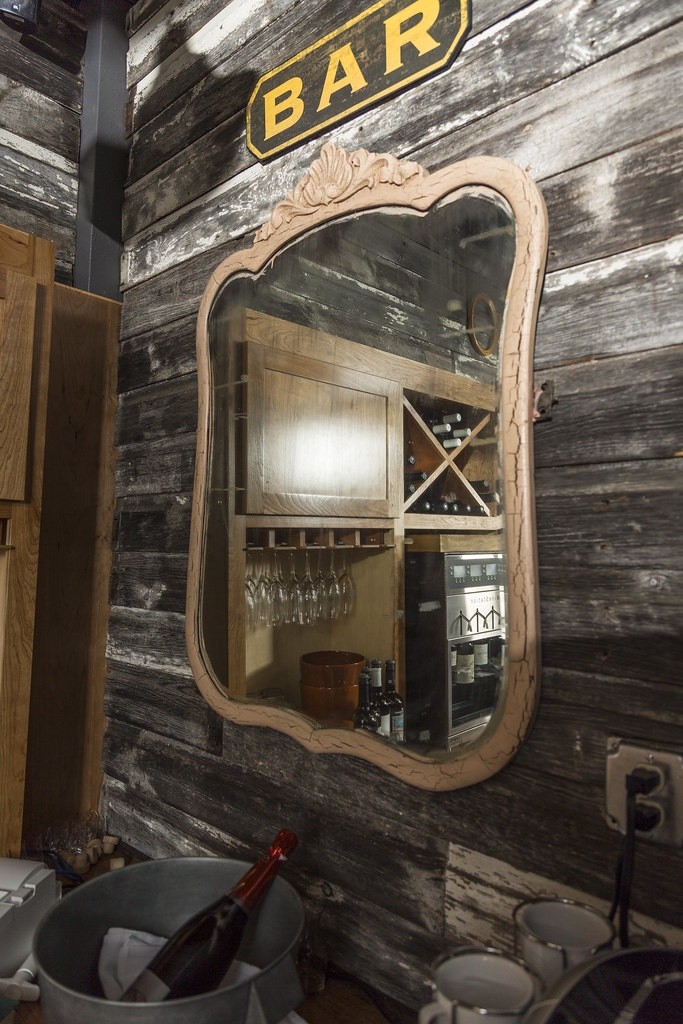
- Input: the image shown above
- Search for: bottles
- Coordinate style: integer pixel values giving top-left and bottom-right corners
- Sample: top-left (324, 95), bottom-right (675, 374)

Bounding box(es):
top-left (450, 638), bottom-right (505, 684)
top-left (117, 827), bottom-right (299, 1002)
top-left (404, 409), bottom-right (499, 516)
top-left (353, 659), bottom-right (405, 746)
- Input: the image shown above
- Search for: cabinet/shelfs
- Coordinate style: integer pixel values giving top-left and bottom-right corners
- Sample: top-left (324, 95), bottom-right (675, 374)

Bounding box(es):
top-left (0, 222), bottom-right (124, 862)
top-left (224, 299), bottom-right (502, 755)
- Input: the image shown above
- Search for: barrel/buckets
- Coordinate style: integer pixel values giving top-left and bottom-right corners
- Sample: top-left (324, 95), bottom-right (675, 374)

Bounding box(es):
top-left (33, 855), bottom-right (303, 1024)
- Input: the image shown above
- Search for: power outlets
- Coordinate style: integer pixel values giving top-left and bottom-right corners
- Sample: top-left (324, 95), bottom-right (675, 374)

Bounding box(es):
top-left (602, 736), bottom-right (683, 850)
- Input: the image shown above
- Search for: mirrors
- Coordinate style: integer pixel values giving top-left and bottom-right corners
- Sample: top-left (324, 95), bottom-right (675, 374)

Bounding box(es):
top-left (182, 136), bottom-right (551, 801)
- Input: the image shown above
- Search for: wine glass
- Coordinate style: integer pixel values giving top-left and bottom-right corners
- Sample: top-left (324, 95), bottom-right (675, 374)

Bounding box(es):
top-left (245, 550), bottom-right (355, 633)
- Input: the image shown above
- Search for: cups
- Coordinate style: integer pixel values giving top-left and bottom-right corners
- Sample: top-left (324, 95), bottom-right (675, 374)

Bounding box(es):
top-left (512, 896), bottom-right (617, 999)
top-left (300, 651), bottom-right (365, 728)
top-left (418, 947), bottom-right (543, 1024)
